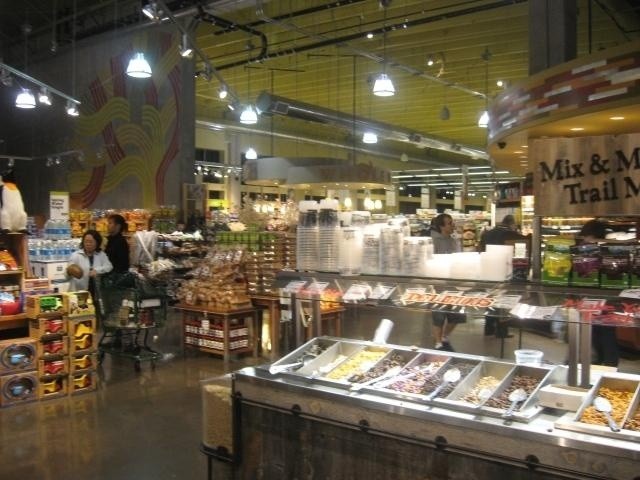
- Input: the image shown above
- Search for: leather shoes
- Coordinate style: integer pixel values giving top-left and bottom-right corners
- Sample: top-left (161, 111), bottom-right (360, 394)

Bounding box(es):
top-left (101, 341), bottom-right (122, 349)
top-left (497, 332), bottom-right (514, 339)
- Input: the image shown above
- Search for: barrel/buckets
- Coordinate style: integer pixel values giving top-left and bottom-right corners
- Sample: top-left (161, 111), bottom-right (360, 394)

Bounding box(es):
top-left (513, 347), bottom-right (544, 367)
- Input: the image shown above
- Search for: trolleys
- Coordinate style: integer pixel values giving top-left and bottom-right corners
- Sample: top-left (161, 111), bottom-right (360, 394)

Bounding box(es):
top-left (88, 267), bottom-right (167, 372)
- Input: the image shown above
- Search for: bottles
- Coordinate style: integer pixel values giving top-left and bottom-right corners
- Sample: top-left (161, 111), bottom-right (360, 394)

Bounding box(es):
top-left (27, 218), bottom-right (82, 262)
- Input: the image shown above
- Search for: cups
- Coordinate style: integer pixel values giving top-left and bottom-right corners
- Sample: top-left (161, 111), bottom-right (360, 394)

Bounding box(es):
top-left (513, 349), bottom-right (544, 365)
top-left (297, 199), bottom-right (362, 277)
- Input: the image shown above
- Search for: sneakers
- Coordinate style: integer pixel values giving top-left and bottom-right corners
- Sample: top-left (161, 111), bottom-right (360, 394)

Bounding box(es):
top-left (434, 345), bottom-right (448, 351)
top-left (442, 342), bottom-right (454, 351)
top-left (591, 360), bottom-right (603, 365)
top-left (601, 360), bottom-right (618, 367)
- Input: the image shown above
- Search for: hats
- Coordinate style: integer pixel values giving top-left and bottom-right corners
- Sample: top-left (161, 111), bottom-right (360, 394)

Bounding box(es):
top-left (577, 221), bottom-right (605, 235)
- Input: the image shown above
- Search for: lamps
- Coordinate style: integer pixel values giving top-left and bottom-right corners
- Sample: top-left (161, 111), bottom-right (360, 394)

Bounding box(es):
top-left (478, 44), bottom-right (492, 128)
top-left (372, 2), bottom-right (396, 98)
top-left (15, 77), bottom-right (80, 116)
top-left (124, 0), bottom-right (258, 124)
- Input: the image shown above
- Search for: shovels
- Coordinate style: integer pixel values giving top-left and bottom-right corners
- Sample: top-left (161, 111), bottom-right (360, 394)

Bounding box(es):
top-left (594, 396), bottom-right (621, 432)
top-left (422, 367), bottom-right (461, 402)
top-left (350, 365), bottom-right (401, 391)
top-left (501, 388), bottom-right (527, 417)
top-left (474, 388), bottom-right (492, 409)
top-left (268, 353), bottom-right (315, 375)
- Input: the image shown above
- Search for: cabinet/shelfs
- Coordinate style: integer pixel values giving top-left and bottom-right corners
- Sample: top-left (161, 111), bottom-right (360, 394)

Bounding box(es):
top-left (0, 231), bottom-right (32, 330)
top-left (154, 223), bottom-right (209, 320)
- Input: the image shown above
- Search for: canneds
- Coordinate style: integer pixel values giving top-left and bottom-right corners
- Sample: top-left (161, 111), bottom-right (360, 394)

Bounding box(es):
top-left (214, 224), bottom-right (276, 251)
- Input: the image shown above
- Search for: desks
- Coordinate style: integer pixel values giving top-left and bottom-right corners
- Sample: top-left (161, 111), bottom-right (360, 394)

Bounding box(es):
top-left (171, 294), bottom-right (347, 363)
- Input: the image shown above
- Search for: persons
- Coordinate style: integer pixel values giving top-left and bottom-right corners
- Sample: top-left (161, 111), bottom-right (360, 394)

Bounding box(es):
top-left (475, 215), bottom-right (531, 337)
top-left (134, 224), bottom-right (156, 274)
top-left (66, 228), bottom-right (113, 318)
top-left (427, 210), bottom-right (466, 352)
top-left (566, 216), bottom-right (619, 367)
top-left (105, 214), bottom-right (132, 276)
